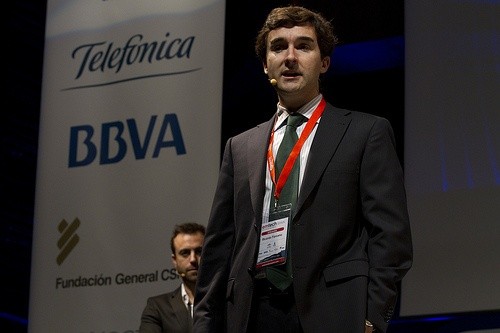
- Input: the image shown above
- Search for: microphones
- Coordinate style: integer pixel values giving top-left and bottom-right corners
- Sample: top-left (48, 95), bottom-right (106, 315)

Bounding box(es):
top-left (268, 79), bottom-right (277, 85)
top-left (176, 270), bottom-right (186, 277)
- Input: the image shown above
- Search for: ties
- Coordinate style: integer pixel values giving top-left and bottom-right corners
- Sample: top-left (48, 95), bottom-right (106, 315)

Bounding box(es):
top-left (265, 113), bottom-right (305, 290)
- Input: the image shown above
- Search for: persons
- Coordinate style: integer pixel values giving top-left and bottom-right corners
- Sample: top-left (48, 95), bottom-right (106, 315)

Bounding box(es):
top-left (136, 222), bottom-right (207, 332)
top-left (191, 5), bottom-right (414, 332)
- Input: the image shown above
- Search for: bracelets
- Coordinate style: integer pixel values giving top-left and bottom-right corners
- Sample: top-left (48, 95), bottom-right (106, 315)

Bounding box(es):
top-left (364, 320), bottom-right (374, 327)
